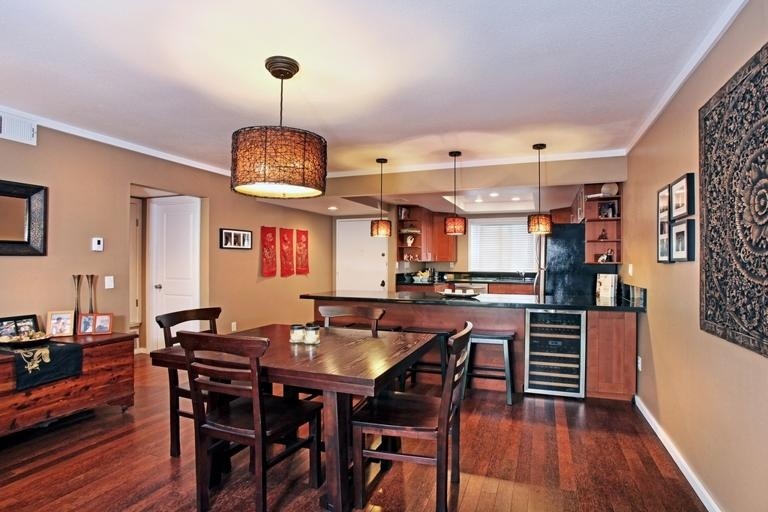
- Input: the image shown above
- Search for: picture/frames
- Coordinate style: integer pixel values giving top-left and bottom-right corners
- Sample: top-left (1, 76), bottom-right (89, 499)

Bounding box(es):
top-left (77, 312), bottom-right (99, 336)
top-left (92, 312), bottom-right (112, 334)
top-left (45, 309), bottom-right (75, 337)
top-left (656, 170), bottom-right (695, 265)
top-left (0, 314), bottom-right (40, 342)
top-left (219, 228), bottom-right (253, 250)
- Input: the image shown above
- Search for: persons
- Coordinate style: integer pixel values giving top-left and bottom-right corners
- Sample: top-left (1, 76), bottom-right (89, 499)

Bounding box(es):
top-left (52, 317), bottom-right (66, 334)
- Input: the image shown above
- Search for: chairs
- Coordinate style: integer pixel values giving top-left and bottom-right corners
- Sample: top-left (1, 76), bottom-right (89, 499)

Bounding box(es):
top-left (174, 326), bottom-right (324, 511)
top-left (347, 319), bottom-right (473, 512)
top-left (308, 303), bottom-right (385, 444)
top-left (154, 307), bottom-right (274, 475)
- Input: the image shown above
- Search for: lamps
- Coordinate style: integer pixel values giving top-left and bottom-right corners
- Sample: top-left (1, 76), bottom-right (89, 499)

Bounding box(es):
top-left (370, 158), bottom-right (391, 239)
top-left (226, 52), bottom-right (334, 202)
top-left (526, 141), bottom-right (554, 234)
top-left (445, 149), bottom-right (466, 235)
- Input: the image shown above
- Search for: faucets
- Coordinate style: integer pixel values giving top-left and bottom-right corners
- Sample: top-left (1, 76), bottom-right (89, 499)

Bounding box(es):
top-left (516, 271), bottom-right (526, 281)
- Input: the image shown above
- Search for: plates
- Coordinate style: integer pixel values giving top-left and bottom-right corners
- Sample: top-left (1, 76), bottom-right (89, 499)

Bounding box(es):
top-left (436, 291), bottom-right (480, 298)
top-left (0, 336), bottom-right (51, 349)
top-left (439, 297), bottom-right (480, 302)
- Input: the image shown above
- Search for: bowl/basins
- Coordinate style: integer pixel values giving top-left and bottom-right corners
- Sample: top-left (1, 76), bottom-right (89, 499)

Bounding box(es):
top-left (413, 277), bottom-right (422, 281)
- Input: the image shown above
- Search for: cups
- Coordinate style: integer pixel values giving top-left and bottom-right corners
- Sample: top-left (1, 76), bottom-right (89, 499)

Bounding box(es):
top-left (444, 288), bottom-right (474, 294)
top-left (404, 252), bottom-right (420, 261)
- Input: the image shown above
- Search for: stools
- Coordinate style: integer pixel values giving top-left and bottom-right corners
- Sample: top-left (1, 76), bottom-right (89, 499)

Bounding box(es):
top-left (304, 317), bottom-right (356, 330)
top-left (400, 327), bottom-right (457, 394)
top-left (458, 329), bottom-right (520, 410)
top-left (351, 322), bottom-right (402, 334)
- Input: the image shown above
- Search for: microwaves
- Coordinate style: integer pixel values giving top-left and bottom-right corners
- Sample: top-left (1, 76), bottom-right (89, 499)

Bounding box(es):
top-left (288, 324), bottom-right (304, 342)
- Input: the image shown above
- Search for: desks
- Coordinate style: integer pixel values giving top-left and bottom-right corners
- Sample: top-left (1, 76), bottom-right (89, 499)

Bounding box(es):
top-left (141, 323), bottom-right (443, 512)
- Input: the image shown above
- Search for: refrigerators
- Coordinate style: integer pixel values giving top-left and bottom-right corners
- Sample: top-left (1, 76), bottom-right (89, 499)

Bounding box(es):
top-left (532, 223), bottom-right (617, 294)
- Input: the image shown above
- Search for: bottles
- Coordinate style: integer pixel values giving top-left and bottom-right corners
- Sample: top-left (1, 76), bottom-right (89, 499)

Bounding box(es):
top-left (304, 322), bottom-right (321, 344)
top-left (290, 343), bottom-right (304, 361)
top-left (304, 345), bottom-right (316, 362)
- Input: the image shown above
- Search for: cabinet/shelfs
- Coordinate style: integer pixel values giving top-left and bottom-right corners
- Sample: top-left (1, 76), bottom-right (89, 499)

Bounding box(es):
top-left (583, 181), bottom-right (624, 265)
top-left (395, 203), bottom-right (422, 261)
top-left (0, 326), bottom-right (142, 446)
top-left (422, 209), bottom-right (436, 260)
top-left (436, 211), bottom-right (458, 263)
top-left (569, 183), bottom-right (586, 224)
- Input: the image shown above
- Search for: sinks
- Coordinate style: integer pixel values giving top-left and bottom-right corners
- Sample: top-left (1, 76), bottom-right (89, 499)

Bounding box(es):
top-left (496, 276), bottom-right (525, 281)
